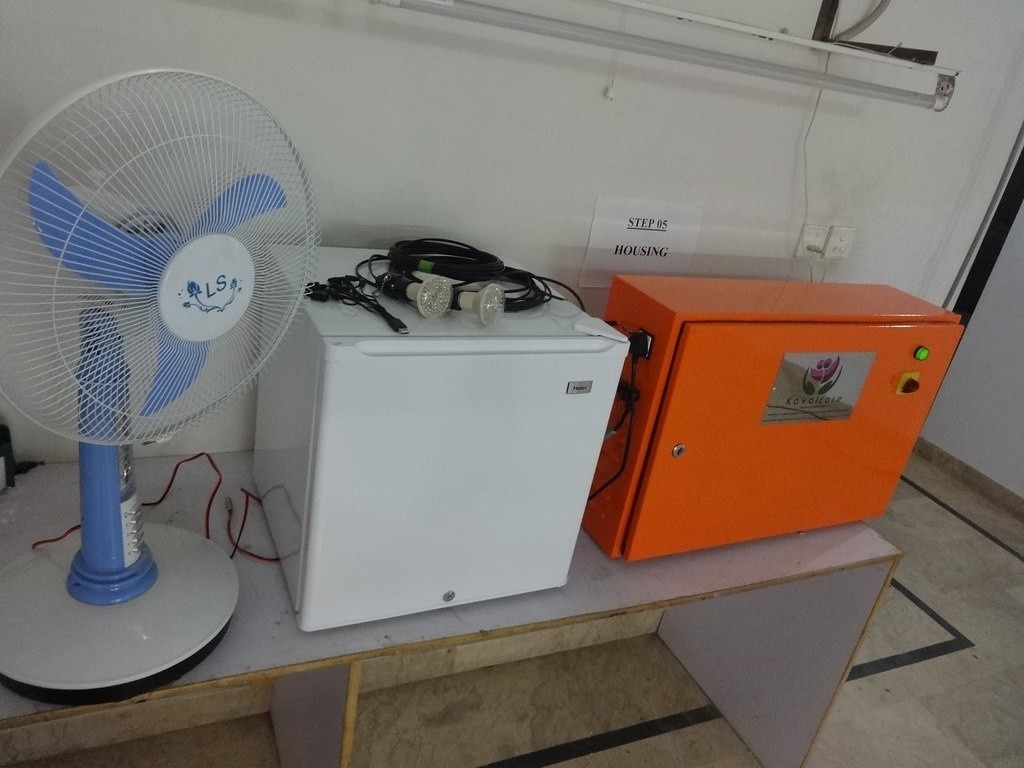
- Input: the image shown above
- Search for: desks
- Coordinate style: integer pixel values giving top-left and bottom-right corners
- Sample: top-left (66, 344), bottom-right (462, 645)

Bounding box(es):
top-left (0, 450), bottom-right (904, 768)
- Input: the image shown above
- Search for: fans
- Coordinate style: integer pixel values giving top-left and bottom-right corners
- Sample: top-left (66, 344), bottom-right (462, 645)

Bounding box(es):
top-left (0, 65), bottom-right (320, 706)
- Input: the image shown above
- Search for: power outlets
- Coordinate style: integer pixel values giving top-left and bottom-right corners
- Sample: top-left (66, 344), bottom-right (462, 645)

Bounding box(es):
top-left (793, 224), bottom-right (827, 258)
